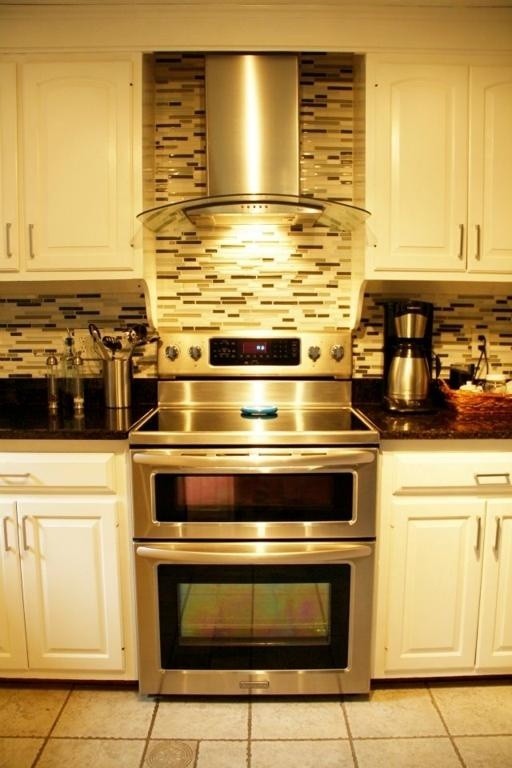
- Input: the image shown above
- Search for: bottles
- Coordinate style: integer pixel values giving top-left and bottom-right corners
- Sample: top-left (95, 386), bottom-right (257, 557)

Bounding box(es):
top-left (484, 373), bottom-right (507, 394)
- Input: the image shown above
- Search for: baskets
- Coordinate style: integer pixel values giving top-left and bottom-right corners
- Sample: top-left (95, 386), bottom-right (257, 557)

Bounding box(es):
top-left (437, 378), bottom-right (512, 414)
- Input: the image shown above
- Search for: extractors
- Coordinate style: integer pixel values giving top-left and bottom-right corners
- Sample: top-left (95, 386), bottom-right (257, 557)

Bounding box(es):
top-left (137, 53), bottom-right (371, 232)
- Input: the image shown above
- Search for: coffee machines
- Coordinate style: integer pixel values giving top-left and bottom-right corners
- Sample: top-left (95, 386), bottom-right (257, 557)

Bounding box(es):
top-left (380, 297), bottom-right (445, 414)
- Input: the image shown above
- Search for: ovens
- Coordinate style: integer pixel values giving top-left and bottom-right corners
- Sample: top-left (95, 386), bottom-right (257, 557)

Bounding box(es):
top-left (128, 448), bottom-right (376, 699)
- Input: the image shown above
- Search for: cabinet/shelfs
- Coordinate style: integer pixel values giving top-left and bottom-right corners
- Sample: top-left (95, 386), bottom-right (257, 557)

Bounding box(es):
top-left (2, 44), bottom-right (142, 282)
top-left (371, 448), bottom-right (512, 687)
top-left (364, 52), bottom-right (509, 286)
top-left (3, 450), bottom-right (138, 685)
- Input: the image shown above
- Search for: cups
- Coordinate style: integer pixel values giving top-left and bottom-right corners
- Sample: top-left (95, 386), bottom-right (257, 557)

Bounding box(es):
top-left (450, 362), bottom-right (475, 387)
top-left (103, 359), bottom-right (131, 409)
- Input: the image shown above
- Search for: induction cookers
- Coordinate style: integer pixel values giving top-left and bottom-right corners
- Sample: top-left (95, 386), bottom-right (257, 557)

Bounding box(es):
top-left (124, 377), bottom-right (380, 447)
